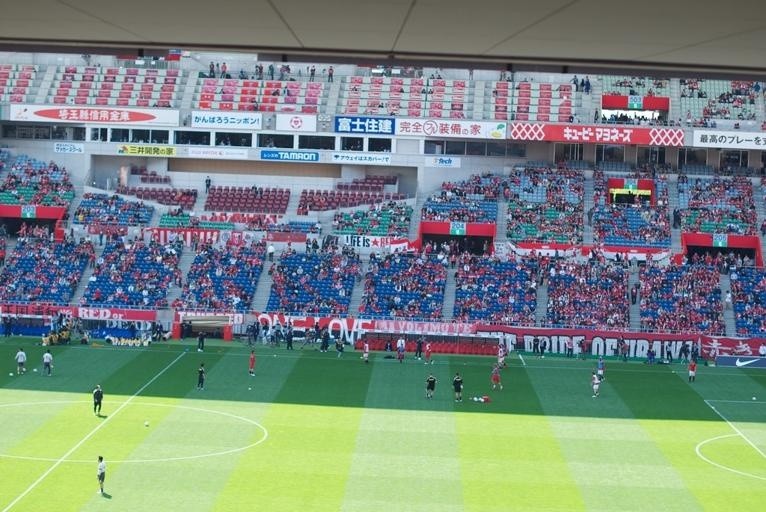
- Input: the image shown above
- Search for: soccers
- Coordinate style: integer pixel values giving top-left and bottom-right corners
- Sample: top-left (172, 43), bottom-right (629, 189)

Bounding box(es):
top-left (145, 421), bottom-right (150, 426)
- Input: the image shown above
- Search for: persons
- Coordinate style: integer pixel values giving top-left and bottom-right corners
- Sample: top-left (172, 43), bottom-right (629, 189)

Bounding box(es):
top-left (91, 384), bottom-right (103, 417)
top-left (589, 372), bottom-right (600, 399)
top-left (450, 377), bottom-right (462, 404)
top-left (94, 456), bottom-right (106, 496)
top-left (424, 373), bottom-right (438, 400)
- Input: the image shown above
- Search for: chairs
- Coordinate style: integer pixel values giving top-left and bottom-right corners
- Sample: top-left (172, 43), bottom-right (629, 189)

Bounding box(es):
top-left (0, 55), bottom-right (765, 345)
